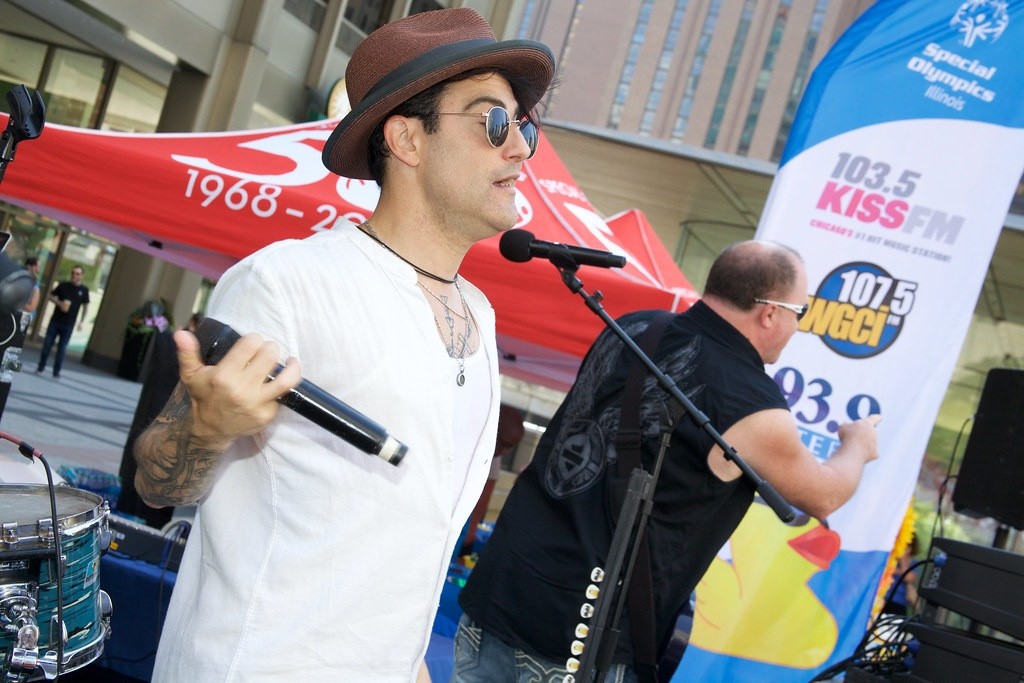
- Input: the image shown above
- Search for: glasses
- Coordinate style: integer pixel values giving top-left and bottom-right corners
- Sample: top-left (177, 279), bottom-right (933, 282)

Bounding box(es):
top-left (411, 106), bottom-right (539, 159)
top-left (755, 298), bottom-right (809, 322)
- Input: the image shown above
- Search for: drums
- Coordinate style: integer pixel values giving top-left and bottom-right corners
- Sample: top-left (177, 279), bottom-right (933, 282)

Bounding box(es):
top-left (0, 480), bottom-right (117, 683)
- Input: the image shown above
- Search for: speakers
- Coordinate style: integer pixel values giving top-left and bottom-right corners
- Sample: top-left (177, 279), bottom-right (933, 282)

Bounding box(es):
top-left (951, 367), bottom-right (1024, 531)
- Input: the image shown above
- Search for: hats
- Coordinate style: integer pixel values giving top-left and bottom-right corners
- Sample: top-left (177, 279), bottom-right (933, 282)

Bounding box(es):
top-left (322, 7), bottom-right (556, 180)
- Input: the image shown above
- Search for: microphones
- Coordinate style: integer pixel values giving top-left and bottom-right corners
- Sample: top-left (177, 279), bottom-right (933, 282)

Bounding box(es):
top-left (191, 318), bottom-right (408, 467)
top-left (499, 228), bottom-right (626, 269)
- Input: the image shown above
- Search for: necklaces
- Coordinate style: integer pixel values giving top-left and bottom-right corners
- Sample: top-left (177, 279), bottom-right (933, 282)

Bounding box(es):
top-left (356, 219), bottom-right (473, 386)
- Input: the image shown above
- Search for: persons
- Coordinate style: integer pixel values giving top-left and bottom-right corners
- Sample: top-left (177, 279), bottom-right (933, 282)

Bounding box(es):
top-left (881, 532), bottom-right (921, 620)
top-left (33, 265), bottom-right (89, 378)
top-left (133, 7), bottom-right (564, 683)
top-left (455, 240), bottom-right (883, 682)
top-left (15, 256), bottom-right (40, 350)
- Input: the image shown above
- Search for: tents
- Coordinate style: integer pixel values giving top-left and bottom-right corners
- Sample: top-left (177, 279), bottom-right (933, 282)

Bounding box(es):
top-left (0, 115), bottom-right (707, 388)
